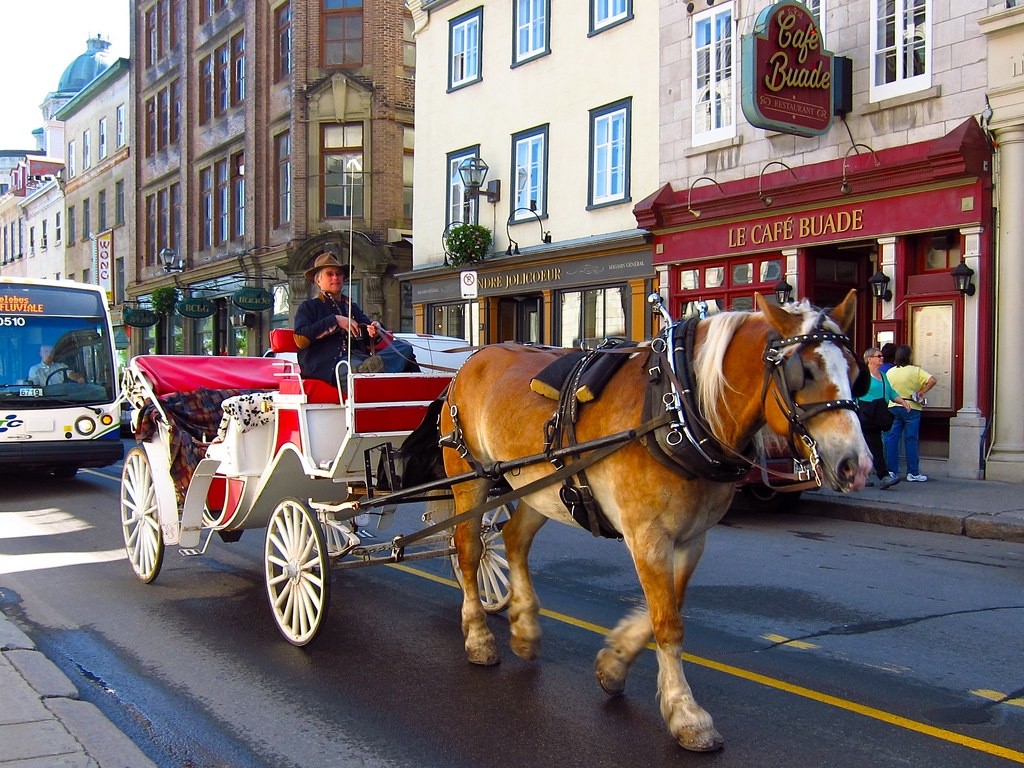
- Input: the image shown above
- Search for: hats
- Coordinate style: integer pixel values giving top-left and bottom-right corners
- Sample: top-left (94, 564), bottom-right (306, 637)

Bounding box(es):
top-left (305, 250), bottom-right (354, 284)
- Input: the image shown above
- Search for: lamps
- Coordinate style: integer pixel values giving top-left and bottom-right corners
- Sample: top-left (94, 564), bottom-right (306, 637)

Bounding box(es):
top-left (158, 247), bottom-right (185, 273)
top-left (688, 177), bottom-right (723, 219)
top-left (839, 143), bottom-right (882, 194)
top-left (458, 157), bottom-right (501, 203)
top-left (950, 256), bottom-right (975, 297)
top-left (757, 161), bottom-right (798, 206)
top-left (504, 208), bottom-right (551, 258)
top-left (774, 278), bottom-right (793, 304)
top-left (868, 268), bottom-right (893, 303)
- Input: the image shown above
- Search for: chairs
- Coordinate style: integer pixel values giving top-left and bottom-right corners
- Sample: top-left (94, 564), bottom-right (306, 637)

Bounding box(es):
top-left (26, 345), bottom-right (68, 385)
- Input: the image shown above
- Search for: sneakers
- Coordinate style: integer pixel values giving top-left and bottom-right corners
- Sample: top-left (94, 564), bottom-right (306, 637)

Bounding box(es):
top-left (907, 472), bottom-right (928, 482)
top-left (890, 471), bottom-right (896, 478)
top-left (865, 478), bottom-right (874, 487)
top-left (879, 476), bottom-right (899, 490)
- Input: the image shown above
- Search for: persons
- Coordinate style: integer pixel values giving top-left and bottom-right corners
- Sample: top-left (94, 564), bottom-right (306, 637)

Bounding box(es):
top-left (857, 343), bottom-right (937, 490)
top-left (28, 344), bottom-right (85, 387)
top-left (292, 252), bottom-right (413, 393)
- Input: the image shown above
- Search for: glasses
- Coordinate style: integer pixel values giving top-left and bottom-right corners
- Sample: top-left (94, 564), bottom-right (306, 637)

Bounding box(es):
top-left (868, 355), bottom-right (883, 358)
top-left (321, 270), bottom-right (345, 277)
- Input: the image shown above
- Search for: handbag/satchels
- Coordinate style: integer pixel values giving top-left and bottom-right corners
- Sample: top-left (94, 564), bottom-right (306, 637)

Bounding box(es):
top-left (872, 396), bottom-right (895, 431)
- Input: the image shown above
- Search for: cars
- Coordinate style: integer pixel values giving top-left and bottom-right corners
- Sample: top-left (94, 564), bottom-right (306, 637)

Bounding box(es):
top-left (120, 400), bottom-right (134, 436)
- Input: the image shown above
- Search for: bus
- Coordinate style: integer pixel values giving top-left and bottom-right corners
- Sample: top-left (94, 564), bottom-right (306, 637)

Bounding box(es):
top-left (0, 276), bottom-right (124, 480)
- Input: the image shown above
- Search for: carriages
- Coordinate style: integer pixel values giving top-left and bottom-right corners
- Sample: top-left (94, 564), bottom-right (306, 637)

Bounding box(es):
top-left (121, 288), bottom-right (873, 752)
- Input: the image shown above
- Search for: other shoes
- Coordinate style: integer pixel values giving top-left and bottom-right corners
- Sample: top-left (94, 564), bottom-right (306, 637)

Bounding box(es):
top-left (354, 355), bottom-right (385, 374)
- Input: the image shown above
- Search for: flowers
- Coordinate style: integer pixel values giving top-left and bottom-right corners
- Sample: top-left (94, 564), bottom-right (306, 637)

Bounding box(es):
top-left (446, 223), bottom-right (493, 263)
top-left (152, 287), bottom-right (177, 313)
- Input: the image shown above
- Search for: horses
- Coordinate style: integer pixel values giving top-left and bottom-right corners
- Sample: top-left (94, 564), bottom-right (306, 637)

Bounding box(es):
top-left (437, 291), bottom-right (875, 751)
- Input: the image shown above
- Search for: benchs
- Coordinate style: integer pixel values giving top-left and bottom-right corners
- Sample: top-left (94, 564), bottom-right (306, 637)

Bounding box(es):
top-left (136, 354), bottom-right (290, 388)
